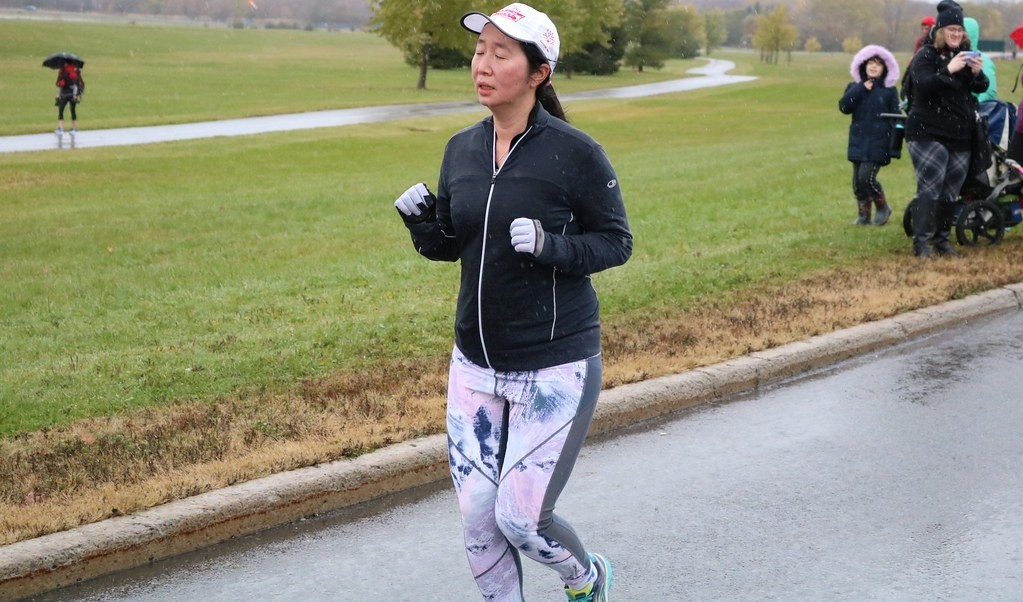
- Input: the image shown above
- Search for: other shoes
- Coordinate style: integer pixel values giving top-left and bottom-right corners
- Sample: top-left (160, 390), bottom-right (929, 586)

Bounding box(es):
top-left (69, 128), bottom-right (76, 133)
top-left (55, 128), bottom-right (63, 135)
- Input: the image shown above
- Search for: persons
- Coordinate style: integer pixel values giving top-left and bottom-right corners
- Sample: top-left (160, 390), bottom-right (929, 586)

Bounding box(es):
top-left (395, 2), bottom-right (633, 602)
top-left (54, 62), bottom-right (85, 135)
top-left (838, 43), bottom-right (905, 228)
top-left (900, 0), bottom-right (1023, 259)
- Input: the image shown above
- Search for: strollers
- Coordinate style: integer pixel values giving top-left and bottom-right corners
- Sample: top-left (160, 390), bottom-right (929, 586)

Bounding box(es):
top-left (877, 100), bottom-right (1022, 248)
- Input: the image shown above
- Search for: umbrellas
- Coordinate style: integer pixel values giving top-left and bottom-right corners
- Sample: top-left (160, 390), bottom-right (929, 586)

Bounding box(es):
top-left (1009, 23), bottom-right (1023, 48)
top-left (42, 52), bottom-right (85, 69)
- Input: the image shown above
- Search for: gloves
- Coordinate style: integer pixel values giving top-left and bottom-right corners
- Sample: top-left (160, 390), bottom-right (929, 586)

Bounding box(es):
top-left (509, 218), bottom-right (552, 264)
top-left (395, 183), bottom-right (437, 233)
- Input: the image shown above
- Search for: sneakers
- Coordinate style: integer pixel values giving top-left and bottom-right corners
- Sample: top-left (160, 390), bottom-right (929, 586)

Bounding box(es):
top-left (564, 552), bottom-right (616, 602)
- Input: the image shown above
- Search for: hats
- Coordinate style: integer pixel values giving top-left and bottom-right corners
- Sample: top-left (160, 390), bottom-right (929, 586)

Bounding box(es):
top-left (933, 0), bottom-right (966, 31)
top-left (459, 3), bottom-right (562, 76)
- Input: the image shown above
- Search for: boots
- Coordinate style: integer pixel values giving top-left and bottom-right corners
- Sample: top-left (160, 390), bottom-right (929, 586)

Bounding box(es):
top-left (913, 198), bottom-right (959, 258)
top-left (850, 194), bottom-right (892, 226)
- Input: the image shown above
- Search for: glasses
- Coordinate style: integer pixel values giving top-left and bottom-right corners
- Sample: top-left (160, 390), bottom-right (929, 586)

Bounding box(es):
top-left (941, 26), bottom-right (963, 33)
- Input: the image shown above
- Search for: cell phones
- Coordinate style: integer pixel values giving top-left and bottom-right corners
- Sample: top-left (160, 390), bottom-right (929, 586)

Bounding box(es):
top-left (961, 51), bottom-right (981, 63)
